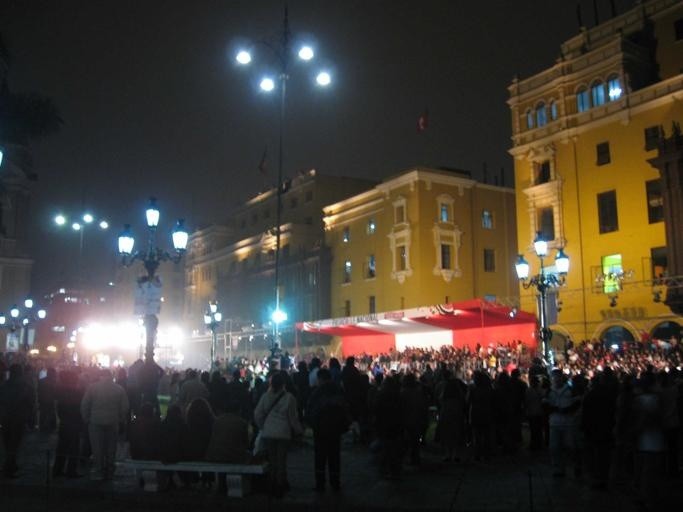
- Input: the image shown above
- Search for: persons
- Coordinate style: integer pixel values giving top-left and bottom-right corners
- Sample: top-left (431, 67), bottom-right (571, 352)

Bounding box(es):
top-left (2, 330), bottom-right (682, 498)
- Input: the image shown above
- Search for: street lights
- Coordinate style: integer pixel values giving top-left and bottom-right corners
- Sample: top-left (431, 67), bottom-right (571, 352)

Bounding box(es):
top-left (55, 187), bottom-right (108, 365)
top-left (0, 294), bottom-right (47, 354)
top-left (233, 2), bottom-right (330, 358)
top-left (118, 194), bottom-right (189, 360)
top-left (204, 296), bottom-right (222, 359)
top-left (515, 234), bottom-right (568, 354)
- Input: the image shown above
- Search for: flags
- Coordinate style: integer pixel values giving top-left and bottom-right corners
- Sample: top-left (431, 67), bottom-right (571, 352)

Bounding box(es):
top-left (417, 111), bottom-right (428, 133)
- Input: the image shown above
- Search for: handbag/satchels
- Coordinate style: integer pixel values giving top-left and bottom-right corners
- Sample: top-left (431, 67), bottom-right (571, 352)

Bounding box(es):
top-left (263, 390), bottom-right (292, 439)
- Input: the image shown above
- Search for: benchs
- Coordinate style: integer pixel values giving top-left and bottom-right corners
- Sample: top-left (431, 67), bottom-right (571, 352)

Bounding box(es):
top-left (113, 458), bottom-right (261, 498)
